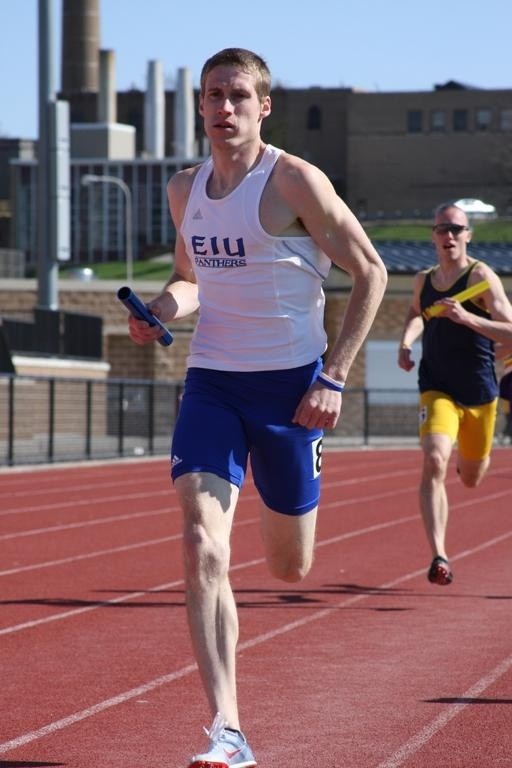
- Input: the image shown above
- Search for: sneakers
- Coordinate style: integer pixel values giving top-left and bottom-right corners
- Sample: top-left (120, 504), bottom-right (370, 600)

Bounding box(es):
top-left (428, 556), bottom-right (453, 585)
top-left (188, 711), bottom-right (258, 767)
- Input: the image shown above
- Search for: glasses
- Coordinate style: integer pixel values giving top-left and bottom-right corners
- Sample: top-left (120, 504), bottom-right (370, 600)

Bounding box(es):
top-left (432, 223), bottom-right (469, 235)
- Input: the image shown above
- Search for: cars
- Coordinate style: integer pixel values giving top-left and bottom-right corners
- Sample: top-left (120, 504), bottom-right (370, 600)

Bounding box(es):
top-left (441, 196), bottom-right (501, 221)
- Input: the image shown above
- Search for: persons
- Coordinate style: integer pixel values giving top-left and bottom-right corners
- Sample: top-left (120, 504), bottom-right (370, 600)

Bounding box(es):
top-left (394, 203), bottom-right (511, 587)
top-left (490, 337), bottom-right (511, 447)
top-left (128, 43), bottom-right (388, 768)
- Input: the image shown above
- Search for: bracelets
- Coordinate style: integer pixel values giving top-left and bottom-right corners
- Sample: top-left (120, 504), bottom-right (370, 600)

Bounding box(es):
top-left (315, 370), bottom-right (346, 393)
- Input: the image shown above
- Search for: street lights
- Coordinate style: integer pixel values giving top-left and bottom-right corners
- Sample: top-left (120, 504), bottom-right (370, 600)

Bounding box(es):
top-left (79, 172), bottom-right (135, 287)
